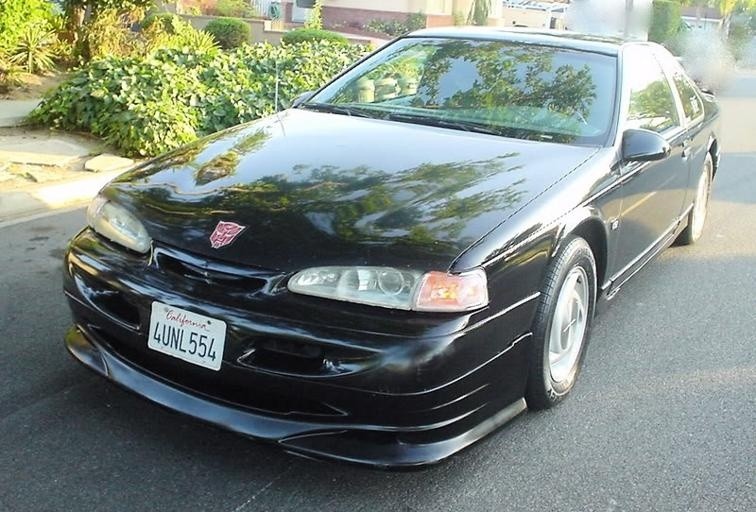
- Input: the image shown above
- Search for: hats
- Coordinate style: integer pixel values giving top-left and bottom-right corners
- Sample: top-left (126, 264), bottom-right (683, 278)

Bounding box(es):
top-left (61, 26), bottom-right (724, 472)
